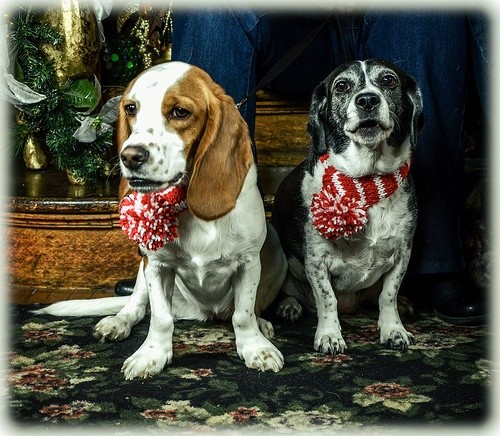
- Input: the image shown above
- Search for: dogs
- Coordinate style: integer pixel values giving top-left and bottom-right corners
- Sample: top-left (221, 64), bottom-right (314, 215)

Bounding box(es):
top-left (270, 58), bottom-right (424, 357)
top-left (27, 60), bottom-right (289, 381)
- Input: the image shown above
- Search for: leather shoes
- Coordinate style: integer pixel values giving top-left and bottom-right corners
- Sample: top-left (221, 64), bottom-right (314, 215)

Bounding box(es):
top-left (435, 293), bottom-right (487, 324)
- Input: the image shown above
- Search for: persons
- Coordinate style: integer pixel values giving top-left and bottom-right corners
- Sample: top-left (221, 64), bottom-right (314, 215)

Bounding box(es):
top-left (116, 1), bottom-right (491, 326)
